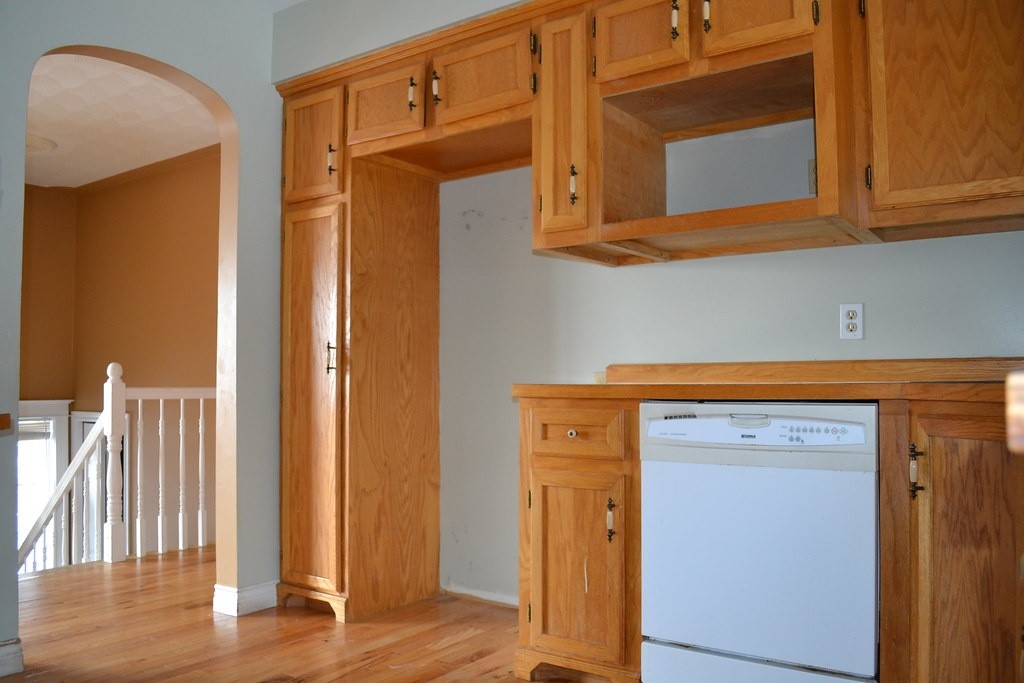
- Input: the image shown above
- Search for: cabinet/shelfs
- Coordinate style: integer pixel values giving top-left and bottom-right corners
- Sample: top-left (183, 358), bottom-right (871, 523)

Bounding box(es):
top-left (509, 357), bottom-right (1024, 683)
top-left (272, 0), bottom-right (1024, 624)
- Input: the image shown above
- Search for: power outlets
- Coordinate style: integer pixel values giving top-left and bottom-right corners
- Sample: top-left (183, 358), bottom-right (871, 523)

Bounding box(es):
top-left (839, 302), bottom-right (864, 339)
